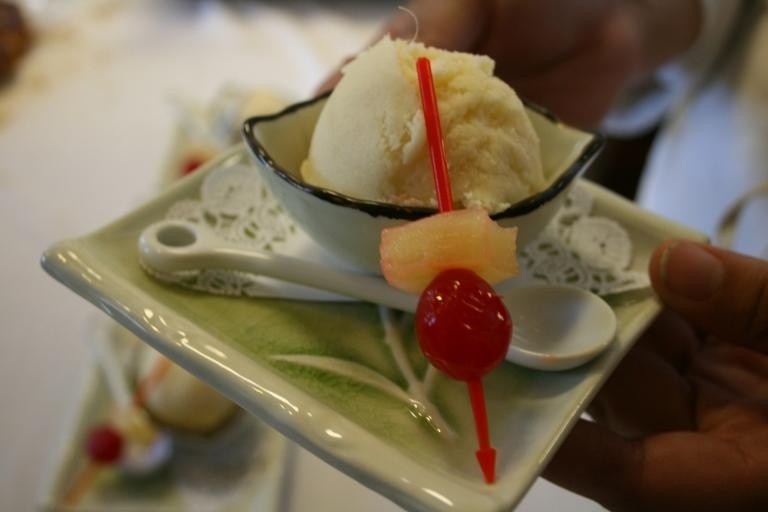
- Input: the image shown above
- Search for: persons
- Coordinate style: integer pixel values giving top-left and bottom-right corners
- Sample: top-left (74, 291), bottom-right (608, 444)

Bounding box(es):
top-left (305, 0), bottom-right (766, 512)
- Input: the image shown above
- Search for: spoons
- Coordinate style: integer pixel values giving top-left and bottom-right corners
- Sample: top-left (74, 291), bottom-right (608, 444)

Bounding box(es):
top-left (136, 216), bottom-right (619, 374)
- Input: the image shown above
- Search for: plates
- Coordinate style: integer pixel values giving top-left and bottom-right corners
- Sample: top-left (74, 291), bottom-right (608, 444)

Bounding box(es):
top-left (38, 137), bottom-right (710, 512)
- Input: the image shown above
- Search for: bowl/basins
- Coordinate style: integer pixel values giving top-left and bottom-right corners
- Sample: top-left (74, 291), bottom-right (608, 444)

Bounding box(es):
top-left (238, 87), bottom-right (610, 278)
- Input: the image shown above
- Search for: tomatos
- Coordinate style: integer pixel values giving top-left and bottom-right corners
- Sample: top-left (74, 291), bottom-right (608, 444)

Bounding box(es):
top-left (415, 267), bottom-right (514, 382)
